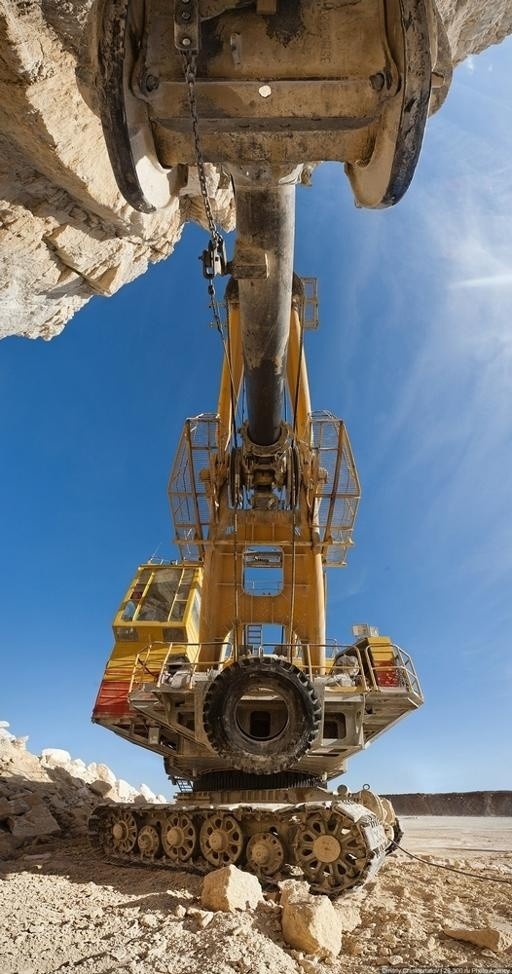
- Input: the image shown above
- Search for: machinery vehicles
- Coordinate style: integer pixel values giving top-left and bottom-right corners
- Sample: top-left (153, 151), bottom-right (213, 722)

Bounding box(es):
top-left (76, 0), bottom-right (453, 896)
